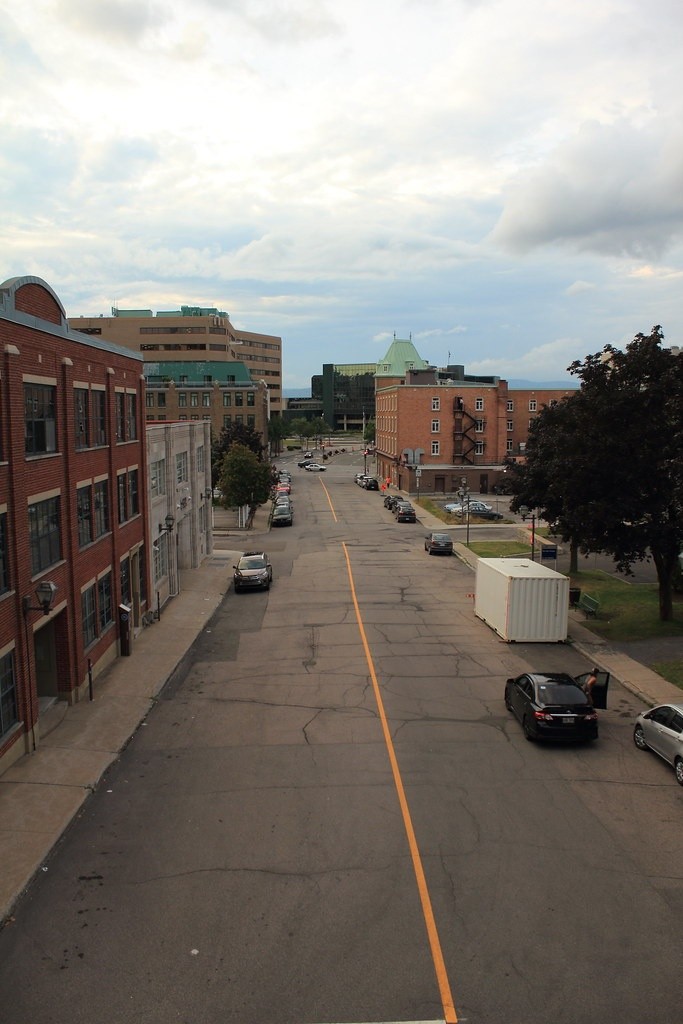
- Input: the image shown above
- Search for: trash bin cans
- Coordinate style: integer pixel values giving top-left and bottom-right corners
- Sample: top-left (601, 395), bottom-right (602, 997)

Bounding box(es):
top-left (569, 588), bottom-right (581, 607)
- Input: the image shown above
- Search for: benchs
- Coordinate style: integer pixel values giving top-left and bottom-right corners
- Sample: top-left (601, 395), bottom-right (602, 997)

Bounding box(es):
top-left (574, 592), bottom-right (600, 620)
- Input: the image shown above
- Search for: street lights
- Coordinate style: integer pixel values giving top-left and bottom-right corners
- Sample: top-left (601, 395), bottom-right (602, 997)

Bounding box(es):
top-left (457, 486), bottom-right (468, 547)
top-left (518, 504), bottom-right (535, 561)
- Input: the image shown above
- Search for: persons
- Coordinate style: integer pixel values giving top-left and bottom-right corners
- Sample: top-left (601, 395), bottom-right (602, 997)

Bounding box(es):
top-left (582, 668), bottom-right (599, 706)
top-left (386, 477), bottom-right (391, 488)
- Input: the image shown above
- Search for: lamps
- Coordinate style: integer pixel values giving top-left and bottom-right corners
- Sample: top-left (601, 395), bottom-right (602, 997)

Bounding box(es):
top-left (23, 580), bottom-right (59, 619)
top-left (200, 487), bottom-right (212, 501)
top-left (159, 514), bottom-right (175, 533)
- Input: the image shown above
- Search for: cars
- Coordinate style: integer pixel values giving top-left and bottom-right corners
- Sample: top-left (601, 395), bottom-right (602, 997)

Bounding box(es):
top-left (392, 501), bottom-right (411, 513)
top-left (395, 506), bottom-right (417, 523)
top-left (304, 452), bottom-right (313, 458)
top-left (632, 703), bottom-right (683, 786)
top-left (270, 470), bottom-right (293, 526)
top-left (505, 671), bottom-right (609, 744)
top-left (444, 498), bottom-right (504, 521)
top-left (424, 532), bottom-right (453, 556)
top-left (305, 464), bottom-right (327, 471)
top-left (297, 460), bottom-right (315, 468)
top-left (354, 473), bottom-right (379, 490)
top-left (383, 495), bottom-right (403, 510)
top-left (232, 551), bottom-right (272, 593)
